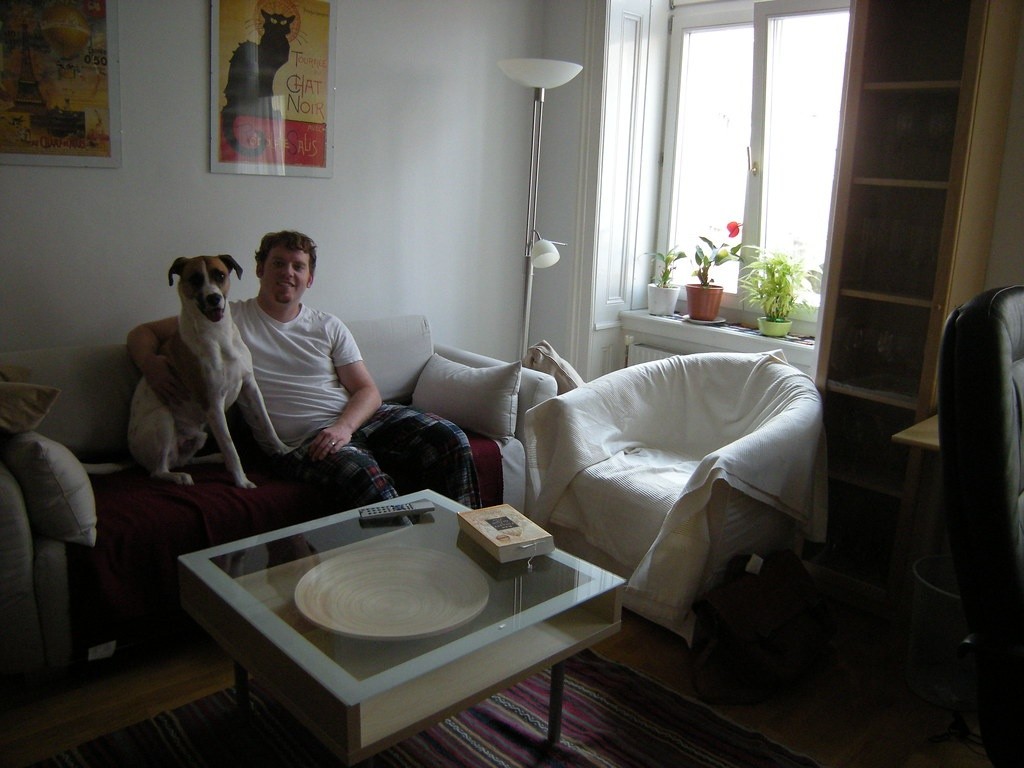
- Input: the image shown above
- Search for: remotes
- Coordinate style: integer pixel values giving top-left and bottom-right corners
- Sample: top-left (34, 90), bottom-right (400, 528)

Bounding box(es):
top-left (359, 502), bottom-right (435, 520)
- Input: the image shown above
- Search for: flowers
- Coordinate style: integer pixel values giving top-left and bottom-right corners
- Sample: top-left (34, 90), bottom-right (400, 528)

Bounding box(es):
top-left (685, 220), bottom-right (744, 289)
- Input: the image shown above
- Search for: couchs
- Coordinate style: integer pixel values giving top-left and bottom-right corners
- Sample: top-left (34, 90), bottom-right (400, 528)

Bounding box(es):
top-left (524, 348), bottom-right (827, 648)
top-left (0, 316), bottom-right (558, 711)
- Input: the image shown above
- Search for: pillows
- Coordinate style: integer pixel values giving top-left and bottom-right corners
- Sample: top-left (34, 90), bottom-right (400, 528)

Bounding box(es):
top-left (413, 353), bottom-right (522, 445)
top-left (0, 382), bottom-right (62, 438)
top-left (522, 341), bottom-right (585, 396)
top-left (3, 430), bottom-right (97, 547)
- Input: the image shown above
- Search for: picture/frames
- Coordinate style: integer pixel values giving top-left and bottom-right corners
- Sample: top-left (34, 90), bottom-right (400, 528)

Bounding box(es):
top-left (0, 1), bottom-right (123, 167)
top-left (211, 0), bottom-right (338, 178)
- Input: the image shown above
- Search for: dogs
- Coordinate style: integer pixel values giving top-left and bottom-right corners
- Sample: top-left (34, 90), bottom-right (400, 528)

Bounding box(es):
top-left (126, 254), bottom-right (300, 491)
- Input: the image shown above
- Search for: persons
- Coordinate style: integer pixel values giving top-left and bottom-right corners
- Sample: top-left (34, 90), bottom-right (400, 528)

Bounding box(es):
top-left (127, 231), bottom-right (483, 530)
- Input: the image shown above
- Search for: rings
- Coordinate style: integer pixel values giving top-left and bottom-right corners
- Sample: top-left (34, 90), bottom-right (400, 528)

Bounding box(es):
top-left (329, 440), bottom-right (335, 446)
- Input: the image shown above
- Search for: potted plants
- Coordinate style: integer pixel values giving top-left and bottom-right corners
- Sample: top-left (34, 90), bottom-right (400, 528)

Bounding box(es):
top-left (738, 245), bottom-right (821, 336)
top-left (646, 245), bottom-right (687, 315)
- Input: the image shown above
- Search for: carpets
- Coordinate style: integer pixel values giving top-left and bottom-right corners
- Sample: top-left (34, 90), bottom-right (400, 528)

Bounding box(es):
top-left (72, 648), bottom-right (827, 768)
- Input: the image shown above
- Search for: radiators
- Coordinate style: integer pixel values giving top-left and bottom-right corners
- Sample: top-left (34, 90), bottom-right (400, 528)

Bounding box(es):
top-left (628, 343), bottom-right (689, 367)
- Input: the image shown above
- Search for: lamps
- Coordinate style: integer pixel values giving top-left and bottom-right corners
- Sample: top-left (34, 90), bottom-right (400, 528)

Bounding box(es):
top-left (496, 58), bottom-right (583, 360)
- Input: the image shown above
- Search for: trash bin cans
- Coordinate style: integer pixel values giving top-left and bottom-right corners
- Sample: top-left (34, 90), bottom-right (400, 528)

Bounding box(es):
top-left (903, 553), bottom-right (975, 714)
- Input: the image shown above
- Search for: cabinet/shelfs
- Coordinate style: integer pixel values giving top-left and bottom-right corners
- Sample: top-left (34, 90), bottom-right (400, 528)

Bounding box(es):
top-left (792, 0), bottom-right (1023, 706)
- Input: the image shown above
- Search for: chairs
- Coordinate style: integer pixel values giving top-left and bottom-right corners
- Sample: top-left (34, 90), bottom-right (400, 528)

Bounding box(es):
top-left (936, 285), bottom-right (1024, 768)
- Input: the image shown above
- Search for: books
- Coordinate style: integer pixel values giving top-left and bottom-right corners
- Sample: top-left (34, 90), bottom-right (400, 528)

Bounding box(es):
top-left (457, 504), bottom-right (555, 564)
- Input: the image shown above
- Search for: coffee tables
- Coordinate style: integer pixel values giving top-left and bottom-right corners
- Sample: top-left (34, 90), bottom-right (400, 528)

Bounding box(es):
top-left (178, 489), bottom-right (626, 768)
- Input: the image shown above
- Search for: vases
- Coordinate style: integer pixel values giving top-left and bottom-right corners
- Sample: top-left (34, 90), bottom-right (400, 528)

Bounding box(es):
top-left (685, 284), bottom-right (723, 321)
